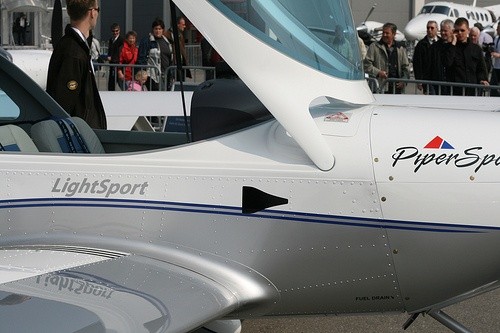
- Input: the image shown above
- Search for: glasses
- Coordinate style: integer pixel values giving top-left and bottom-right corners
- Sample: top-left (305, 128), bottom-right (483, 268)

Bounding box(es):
top-left (426, 27), bottom-right (435, 30)
top-left (453, 29), bottom-right (466, 32)
top-left (88, 7), bottom-right (100, 13)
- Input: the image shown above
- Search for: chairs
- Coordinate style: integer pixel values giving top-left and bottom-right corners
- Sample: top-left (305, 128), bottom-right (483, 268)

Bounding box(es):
top-left (0, 117), bottom-right (105, 154)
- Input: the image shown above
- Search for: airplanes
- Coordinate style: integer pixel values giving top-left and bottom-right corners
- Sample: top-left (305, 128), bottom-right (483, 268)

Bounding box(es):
top-left (306, 5), bottom-right (407, 46)
top-left (0, 0), bottom-right (500, 333)
top-left (404, 1), bottom-right (500, 54)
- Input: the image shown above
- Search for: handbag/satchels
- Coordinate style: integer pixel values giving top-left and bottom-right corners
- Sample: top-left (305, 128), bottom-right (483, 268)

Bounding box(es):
top-left (121, 62), bottom-right (129, 71)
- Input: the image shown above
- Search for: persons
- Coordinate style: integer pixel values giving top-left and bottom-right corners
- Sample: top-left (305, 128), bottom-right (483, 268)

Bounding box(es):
top-left (357, 35), bottom-right (366, 61)
top-left (412, 17), bottom-right (500, 97)
top-left (331, 25), bottom-right (352, 63)
top-left (89, 37), bottom-right (101, 70)
top-left (164, 15), bottom-right (192, 82)
top-left (116, 31), bottom-right (138, 91)
top-left (104, 24), bottom-right (127, 91)
top-left (128, 70), bottom-right (148, 92)
top-left (45, 0), bottom-right (107, 130)
top-left (14, 15), bottom-right (27, 45)
top-left (362, 22), bottom-right (411, 94)
top-left (135, 18), bottom-right (175, 92)
